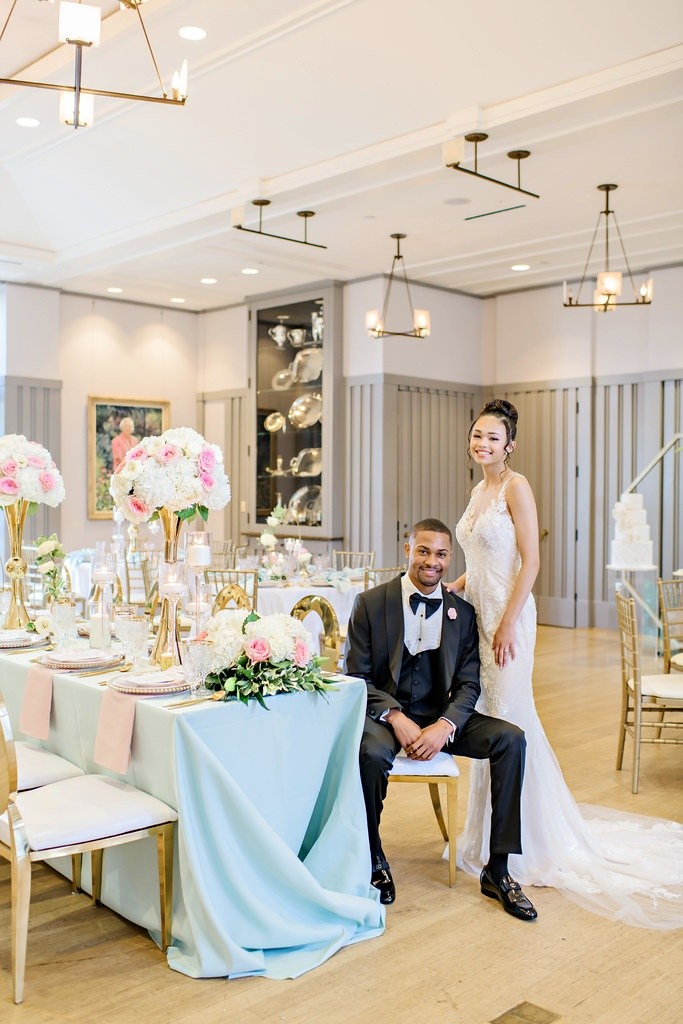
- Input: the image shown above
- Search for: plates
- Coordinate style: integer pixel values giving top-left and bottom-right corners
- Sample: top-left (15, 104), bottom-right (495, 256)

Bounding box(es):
top-left (254, 571), bottom-right (370, 588)
top-left (0, 630), bottom-right (47, 648)
top-left (37, 648), bottom-right (126, 669)
top-left (109, 669), bottom-right (199, 694)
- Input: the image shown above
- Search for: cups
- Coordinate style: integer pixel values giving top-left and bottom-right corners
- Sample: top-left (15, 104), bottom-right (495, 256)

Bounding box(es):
top-left (90, 553), bottom-right (118, 584)
top-left (122, 621), bottom-right (150, 664)
top-left (188, 582), bottom-right (211, 618)
top-left (53, 604), bottom-right (76, 640)
top-left (184, 532), bottom-right (212, 570)
top-left (88, 603), bottom-right (112, 649)
top-left (158, 563), bottom-right (187, 599)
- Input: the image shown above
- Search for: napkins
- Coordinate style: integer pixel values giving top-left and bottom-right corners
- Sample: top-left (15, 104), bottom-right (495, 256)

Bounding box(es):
top-left (93, 693), bottom-right (138, 774)
top-left (20, 666), bottom-right (54, 740)
top-left (41, 638), bottom-right (110, 662)
top-left (133, 665), bottom-right (190, 686)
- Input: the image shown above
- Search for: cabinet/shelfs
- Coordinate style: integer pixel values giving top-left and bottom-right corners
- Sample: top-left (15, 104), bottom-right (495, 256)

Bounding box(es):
top-left (251, 296), bottom-right (325, 529)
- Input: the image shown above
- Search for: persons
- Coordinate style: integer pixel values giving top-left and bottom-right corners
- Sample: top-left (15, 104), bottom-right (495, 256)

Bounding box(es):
top-left (443, 398), bottom-right (577, 888)
top-left (111, 417), bottom-right (139, 474)
top-left (344, 519), bottom-right (538, 922)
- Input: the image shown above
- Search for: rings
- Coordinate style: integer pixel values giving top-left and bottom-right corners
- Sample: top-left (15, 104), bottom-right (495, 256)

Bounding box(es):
top-left (504, 649), bottom-right (509, 652)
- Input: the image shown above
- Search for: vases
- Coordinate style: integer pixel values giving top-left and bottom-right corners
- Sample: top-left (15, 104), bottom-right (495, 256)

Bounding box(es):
top-left (158, 507), bottom-right (187, 563)
top-left (0, 499), bottom-right (33, 631)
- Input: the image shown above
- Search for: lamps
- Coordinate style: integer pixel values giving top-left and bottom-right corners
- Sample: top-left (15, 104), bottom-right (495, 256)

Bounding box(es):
top-left (365, 232), bottom-right (431, 341)
top-left (443, 133), bottom-right (540, 198)
top-left (1, 0), bottom-right (188, 130)
top-left (230, 199), bottom-right (327, 249)
top-left (562, 184), bottom-right (653, 312)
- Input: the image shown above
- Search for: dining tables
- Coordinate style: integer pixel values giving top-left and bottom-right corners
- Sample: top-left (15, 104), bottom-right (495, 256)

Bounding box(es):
top-left (204, 565), bottom-right (379, 645)
top-left (0, 625), bottom-right (386, 979)
top-left (78, 551), bottom-right (220, 604)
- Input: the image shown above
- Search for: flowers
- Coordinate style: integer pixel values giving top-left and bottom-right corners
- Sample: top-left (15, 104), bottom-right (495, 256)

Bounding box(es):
top-left (180, 602), bottom-right (347, 711)
top-left (107, 427), bottom-right (233, 523)
top-left (34, 534), bottom-right (68, 605)
top-left (0, 432), bottom-right (65, 507)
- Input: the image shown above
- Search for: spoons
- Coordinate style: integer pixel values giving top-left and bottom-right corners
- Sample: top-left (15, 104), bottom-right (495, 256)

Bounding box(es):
top-left (165, 690), bottom-right (226, 710)
top-left (79, 663), bottom-right (133, 678)
top-left (9, 643), bottom-right (57, 654)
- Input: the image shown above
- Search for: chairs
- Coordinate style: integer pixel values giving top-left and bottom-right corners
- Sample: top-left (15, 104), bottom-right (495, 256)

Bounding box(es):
top-left (211, 587), bottom-right (253, 615)
top-left (617, 593), bottom-right (683, 795)
top-left (657, 569), bottom-right (683, 675)
top-left (332, 550), bottom-right (408, 590)
top-left (0, 702), bottom-right (177, 1005)
top-left (292, 594), bottom-right (463, 890)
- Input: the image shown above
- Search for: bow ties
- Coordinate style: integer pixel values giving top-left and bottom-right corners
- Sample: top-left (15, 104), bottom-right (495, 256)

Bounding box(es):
top-left (409, 593), bottom-right (441, 618)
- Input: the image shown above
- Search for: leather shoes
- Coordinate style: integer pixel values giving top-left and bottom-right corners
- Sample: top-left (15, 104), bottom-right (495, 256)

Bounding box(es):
top-left (371, 857), bottom-right (396, 905)
top-left (479, 865), bottom-right (537, 921)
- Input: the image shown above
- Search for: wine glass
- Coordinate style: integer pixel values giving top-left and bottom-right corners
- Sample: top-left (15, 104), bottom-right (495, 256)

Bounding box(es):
top-left (186, 639), bottom-right (215, 695)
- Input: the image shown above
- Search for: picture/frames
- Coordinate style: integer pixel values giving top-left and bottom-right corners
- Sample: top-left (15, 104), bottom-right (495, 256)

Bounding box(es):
top-left (87, 395), bottom-right (171, 521)
